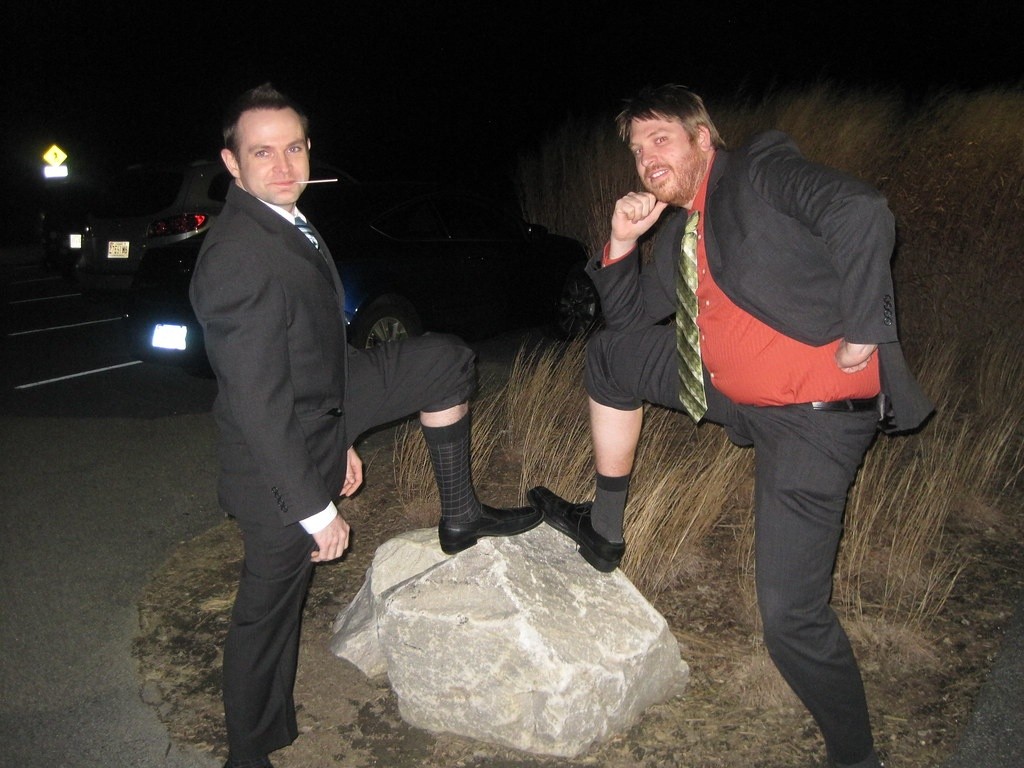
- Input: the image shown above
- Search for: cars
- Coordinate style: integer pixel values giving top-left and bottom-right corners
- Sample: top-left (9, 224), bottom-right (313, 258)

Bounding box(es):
top-left (79, 156), bottom-right (362, 296)
top-left (123, 182), bottom-right (601, 380)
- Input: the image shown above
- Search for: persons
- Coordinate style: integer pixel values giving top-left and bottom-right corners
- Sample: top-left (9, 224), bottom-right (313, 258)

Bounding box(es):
top-left (525, 81), bottom-right (941, 768)
top-left (184, 77), bottom-right (551, 767)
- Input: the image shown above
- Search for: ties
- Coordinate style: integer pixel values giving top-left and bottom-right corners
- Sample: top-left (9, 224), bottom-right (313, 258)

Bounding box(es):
top-left (294, 216), bottom-right (318, 250)
top-left (676, 210), bottom-right (708, 425)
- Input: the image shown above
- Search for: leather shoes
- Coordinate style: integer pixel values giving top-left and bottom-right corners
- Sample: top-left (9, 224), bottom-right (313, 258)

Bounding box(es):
top-left (527, 486), bottom-right (625, 573)
top-left (439, 501), bottom-right (545, 555)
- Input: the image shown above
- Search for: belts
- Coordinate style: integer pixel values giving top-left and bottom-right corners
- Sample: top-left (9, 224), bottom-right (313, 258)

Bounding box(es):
top-left (812, 394), bottom-right (879, 413)
